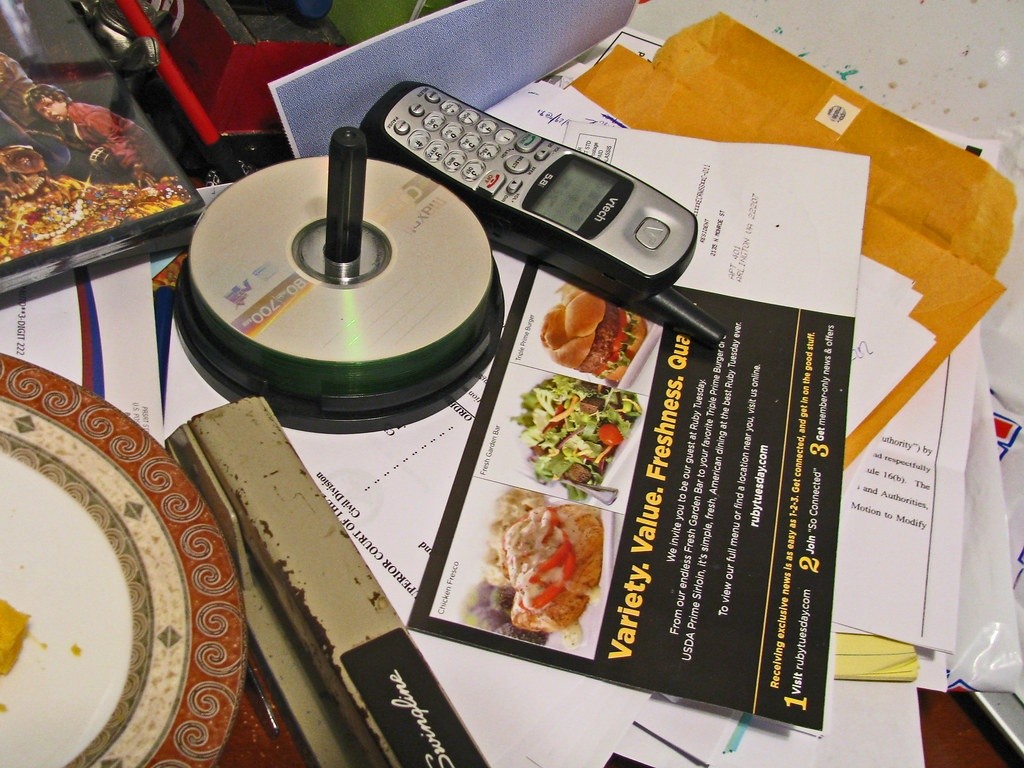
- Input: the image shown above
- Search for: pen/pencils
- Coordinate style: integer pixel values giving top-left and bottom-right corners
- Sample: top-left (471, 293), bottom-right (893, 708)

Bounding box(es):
top-left (115, 1), bottom-right (245, 179)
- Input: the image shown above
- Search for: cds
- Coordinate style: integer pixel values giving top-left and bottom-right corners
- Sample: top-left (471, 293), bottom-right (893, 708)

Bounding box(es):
top-left (189, 152), bottom-right (497, 401)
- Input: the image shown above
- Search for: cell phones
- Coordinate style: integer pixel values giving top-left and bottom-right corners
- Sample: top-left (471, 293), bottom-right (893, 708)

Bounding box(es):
top-left (358, 81), bottom-right (728, 348)
